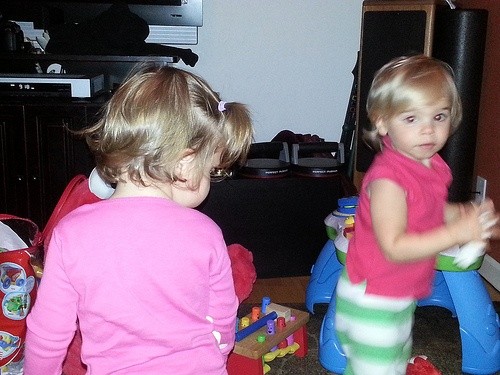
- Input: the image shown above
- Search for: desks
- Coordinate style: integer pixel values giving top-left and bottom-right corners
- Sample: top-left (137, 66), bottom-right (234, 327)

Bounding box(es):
top-left (194, 174), bottom-right (347, 278)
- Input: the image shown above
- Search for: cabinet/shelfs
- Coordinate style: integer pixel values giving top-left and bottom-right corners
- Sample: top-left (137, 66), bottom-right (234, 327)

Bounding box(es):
top-left (0, 50), bottom-right (182, 242)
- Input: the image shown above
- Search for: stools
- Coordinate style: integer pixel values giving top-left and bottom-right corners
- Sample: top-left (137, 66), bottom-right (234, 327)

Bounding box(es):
top-left (226, 303), bottom-right (309, 375)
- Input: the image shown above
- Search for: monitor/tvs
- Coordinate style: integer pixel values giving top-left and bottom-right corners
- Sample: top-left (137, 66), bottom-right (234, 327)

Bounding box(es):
top-left (0, 0), bottom-right (204, 27)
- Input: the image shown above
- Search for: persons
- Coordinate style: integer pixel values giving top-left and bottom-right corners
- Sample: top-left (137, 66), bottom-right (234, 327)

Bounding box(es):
top-left (22, 67), bottom-right (238, 375)
top-left (329, 55), bottom-right (500, 375)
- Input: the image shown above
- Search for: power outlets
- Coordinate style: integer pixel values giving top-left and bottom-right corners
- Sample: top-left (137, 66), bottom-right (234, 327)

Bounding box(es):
top-left (475, 176), bottom-right (486, 203)
